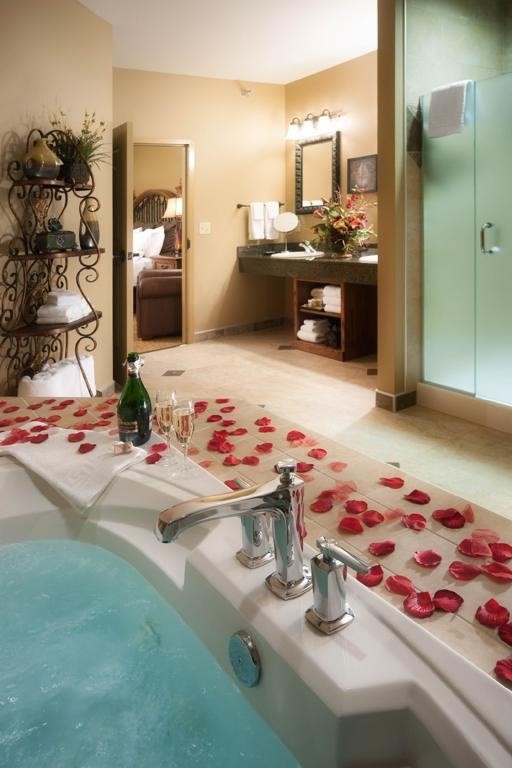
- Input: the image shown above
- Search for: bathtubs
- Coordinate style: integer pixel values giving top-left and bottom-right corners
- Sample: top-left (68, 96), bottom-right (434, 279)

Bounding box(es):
top-left (0, 429), bottom-right (512, 768)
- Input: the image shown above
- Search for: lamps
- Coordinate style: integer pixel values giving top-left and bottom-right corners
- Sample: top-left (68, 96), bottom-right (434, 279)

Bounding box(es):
top-left (161, 197), bottom-right (182, 253)
top-left (284, 110), bottom-right (340, 141)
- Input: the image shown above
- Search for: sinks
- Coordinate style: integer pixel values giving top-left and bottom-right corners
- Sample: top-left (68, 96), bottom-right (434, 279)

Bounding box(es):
top-left (360, 254), bottom-right (378, 263)
top-left (271, 252), bottom-right (325, 258)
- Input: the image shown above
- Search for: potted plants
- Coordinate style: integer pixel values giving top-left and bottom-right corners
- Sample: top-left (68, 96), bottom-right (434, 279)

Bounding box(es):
top-left (48, 110), bottom-right (111, 183)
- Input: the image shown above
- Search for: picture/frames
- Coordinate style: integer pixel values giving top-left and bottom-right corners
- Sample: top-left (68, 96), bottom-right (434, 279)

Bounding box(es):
top-left (347, 155), bottom-right (377, 194)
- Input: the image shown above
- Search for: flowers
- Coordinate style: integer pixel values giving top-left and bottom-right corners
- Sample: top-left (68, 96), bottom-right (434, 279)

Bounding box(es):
top-left (309, 184), bottom-right (377, 253)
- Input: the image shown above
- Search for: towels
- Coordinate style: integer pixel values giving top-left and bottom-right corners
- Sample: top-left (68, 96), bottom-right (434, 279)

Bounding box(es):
top-left (297, 286), bottom-right (341, 344)
top-left (249, 202), bottom-right (265, 241)
top-left (429, 80), bottom-right (472, 138)
top-left (311, 200), bottom-right (321, 206)
top-left (0, 421), bottom-right (147, 516)
top-left (265, 201), bottom-right (280, 240)
top-left (303, 201), bottom-right (311, 206)
top-left (18, 292), bottom-right (97, 397)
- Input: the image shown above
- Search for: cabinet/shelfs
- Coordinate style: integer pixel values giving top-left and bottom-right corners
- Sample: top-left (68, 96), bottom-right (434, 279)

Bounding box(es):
top-left (151, 253), bottom-right (181, 269)
top-left (293, 279), bottom-right (369, 361)
top-left (0, 129), bottom-right (105, 397)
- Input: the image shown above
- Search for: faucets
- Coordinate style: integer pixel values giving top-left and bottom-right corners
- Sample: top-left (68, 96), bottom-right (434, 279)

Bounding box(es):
top-left (155, 460), bottom-right (312, 600)
top-left (299, 241), bottom-right (316, 253)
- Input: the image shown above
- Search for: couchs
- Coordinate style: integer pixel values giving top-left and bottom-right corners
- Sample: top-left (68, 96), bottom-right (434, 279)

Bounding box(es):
top-left (136, 268), bottom-right (182, 340)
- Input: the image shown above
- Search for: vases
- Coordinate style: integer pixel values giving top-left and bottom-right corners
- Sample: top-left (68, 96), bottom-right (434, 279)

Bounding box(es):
top-left (326, 237), bottom-right (351, 258)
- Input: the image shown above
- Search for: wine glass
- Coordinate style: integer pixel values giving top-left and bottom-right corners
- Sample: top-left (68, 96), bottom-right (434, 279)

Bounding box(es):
top-left (155, 387), bottom-right (178, 466)
top-left (171, 398), bottom-right (200, 473)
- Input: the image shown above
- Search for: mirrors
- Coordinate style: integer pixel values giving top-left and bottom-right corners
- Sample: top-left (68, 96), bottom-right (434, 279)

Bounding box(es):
top-left (295, 131), bottom-right (340, 215)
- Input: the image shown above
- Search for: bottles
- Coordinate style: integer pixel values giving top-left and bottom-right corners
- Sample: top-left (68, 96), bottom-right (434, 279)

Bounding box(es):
top-left (118, 351), bottom-right (153, 446)
